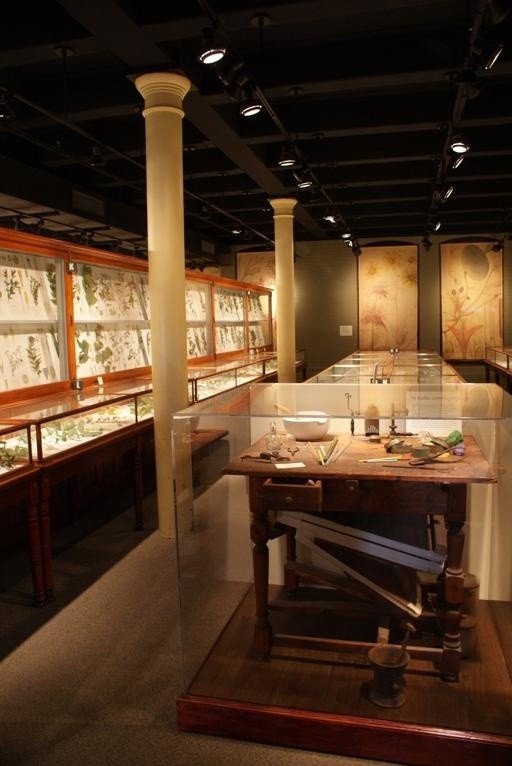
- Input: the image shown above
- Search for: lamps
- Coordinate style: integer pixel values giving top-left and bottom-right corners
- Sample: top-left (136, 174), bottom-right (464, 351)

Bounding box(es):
top-left (278, 146), bottom-right (296, 167)
top-left (231, 222), bottom-right (241, 234)
top-left (198, 28), bottom-right (227, 64)
top-left (90, 146), bottom-right (106, 168)
top-left (237, 85), bottom-right (262, 118)
top-left (426, 215), bottom-right (441, 231)
top-left (451, 135), bottom-right (472, 154)
top-left (325, 215), bottom-right (354, 250)
top-left (445, 153), bottom-right (465, 169)
top-left (470, 38), bottom-right (501, 70)
top-left (435, 180), bottom-right (454, 199)
top-left (296, 169), bottom-right (313, 188)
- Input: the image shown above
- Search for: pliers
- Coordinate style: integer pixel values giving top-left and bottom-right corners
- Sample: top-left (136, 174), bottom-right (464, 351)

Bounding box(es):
top-left (287, 447), bottom-right (299, 456)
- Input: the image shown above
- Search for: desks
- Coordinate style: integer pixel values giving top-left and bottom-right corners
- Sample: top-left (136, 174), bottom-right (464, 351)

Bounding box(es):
top-left (224, 431), bottom-right (501, 682)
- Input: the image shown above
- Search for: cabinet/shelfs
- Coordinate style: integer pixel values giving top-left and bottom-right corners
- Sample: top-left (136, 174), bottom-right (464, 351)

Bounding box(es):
top-left (0, 230), bottom-right (277, 405)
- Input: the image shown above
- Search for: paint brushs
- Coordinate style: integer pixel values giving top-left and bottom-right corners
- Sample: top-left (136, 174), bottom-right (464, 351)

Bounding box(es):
top-left (357, 456), bottom-right (412, 462)
top-left (305, 436), bottom-right (353, 466)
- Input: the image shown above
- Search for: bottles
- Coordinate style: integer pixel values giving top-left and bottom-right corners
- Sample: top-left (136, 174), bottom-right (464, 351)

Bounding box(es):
top-left (264, 421), bottom-right (281, 450)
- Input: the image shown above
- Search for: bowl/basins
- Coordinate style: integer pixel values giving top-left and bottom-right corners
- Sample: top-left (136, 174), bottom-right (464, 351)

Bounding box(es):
top-left (282, 416), bottom-right (330, 440)
